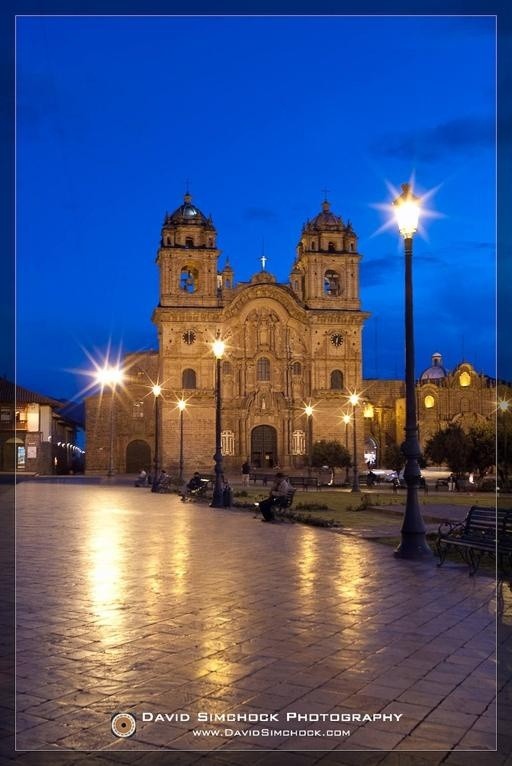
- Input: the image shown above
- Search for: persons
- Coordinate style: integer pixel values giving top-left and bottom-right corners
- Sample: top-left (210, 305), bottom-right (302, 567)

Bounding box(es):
top-left (135, 470), bottom-right (146, 486)
top-left (179, 472), bottom-right (200, 500)
top-left (241, 462), bottom-right (251, 488)
top-left (260, 474), bottom-right (289, 522)
top-left (152, 471), bottom-right (166, 492)
top-left (447, 474), bottom-right (457, 491)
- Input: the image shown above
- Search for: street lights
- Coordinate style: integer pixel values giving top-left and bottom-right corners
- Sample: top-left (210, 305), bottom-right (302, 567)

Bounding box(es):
top-left (95, 363), bottom-right (123, 477)
top-left (208, 340), bottom-right (226, 507)
top-left (343, 415), bottom-right (351, 487)
top-left (349, 393), bottom-right (362, 492)
top-left (151, 384), bottom-right (161, 491)
top-left (305, 406), bottom-right (312, 478)
top-left (177, 400), bottom-right (186, 477)
top-left (393, 180), bottom-right (435, 560)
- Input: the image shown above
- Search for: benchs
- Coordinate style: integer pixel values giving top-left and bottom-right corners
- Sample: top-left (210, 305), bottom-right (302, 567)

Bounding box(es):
top-left (189, 479), bottom-right (209, 502)
top-left (437, 505), bottom-right (512, 577)
top-left (159, 476), bottom-right (170, 492)
top-left (249, 473), bottom-right (321, 492)
top-left (253, 488), bottom-right (296, 523)
top-left (436, 478), bottom-right (458, 492)
top-left (392, 477), bottom-right (428, 495)
